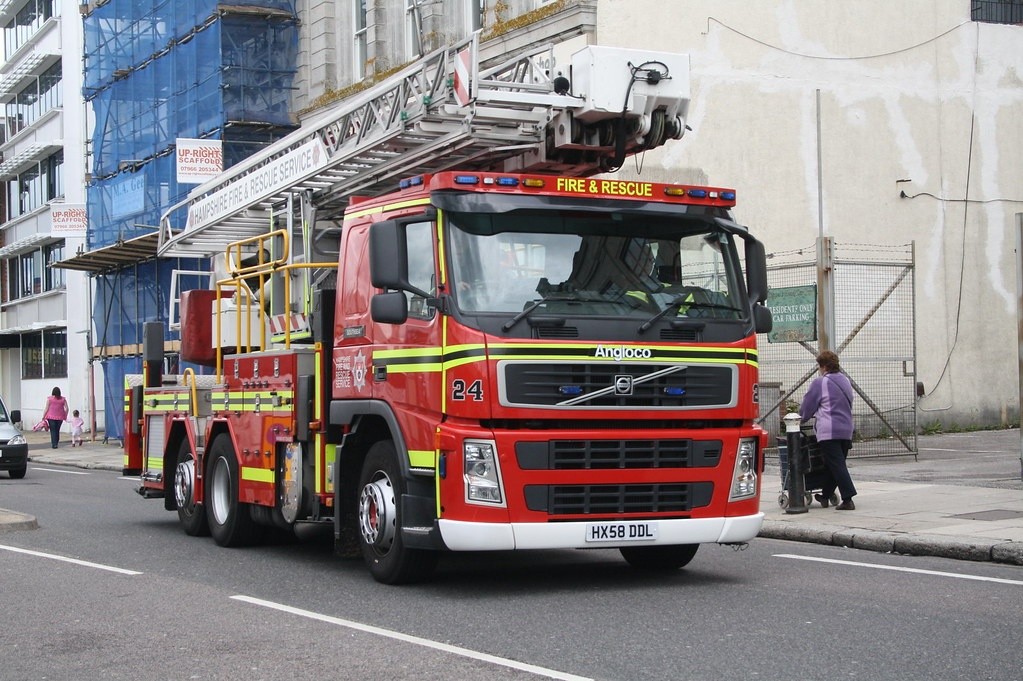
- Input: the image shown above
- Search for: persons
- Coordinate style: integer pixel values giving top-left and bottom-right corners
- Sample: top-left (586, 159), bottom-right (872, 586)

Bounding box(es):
top-left (625, 244), bottom-right (662, 291)
top-left (42, 387), bottom-right (69, 449)
top-left (799, 351), bottom-right (857, 510)
top-left (65, 410), bottom-right (84, 447)
top-left (427, 228), bottom-right (492, 292)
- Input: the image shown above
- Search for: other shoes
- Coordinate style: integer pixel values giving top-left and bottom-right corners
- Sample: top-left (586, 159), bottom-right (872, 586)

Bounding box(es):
top-left (835, 500), bottom-right (855, 509)
top-left (72, 444), bottom-right (75, 447)
top-left (815, 493), bottom-right (828, 507)
top-left (53, 444), bottom-right (58, 448)
top-left (79, 440), bottom-right (82, 445)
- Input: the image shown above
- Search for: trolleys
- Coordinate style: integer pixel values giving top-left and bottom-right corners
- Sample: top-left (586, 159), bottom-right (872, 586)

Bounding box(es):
top-left (775, 415), bottom-right (840, 508)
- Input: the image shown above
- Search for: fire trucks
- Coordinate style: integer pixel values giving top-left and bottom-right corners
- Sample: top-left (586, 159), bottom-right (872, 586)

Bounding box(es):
top-left (122, 29), bottom-right (773, 586)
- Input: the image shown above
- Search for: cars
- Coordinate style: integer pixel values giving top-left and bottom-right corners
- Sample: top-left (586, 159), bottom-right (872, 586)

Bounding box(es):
top-left (0, 398), bottom-right (29, 479)
